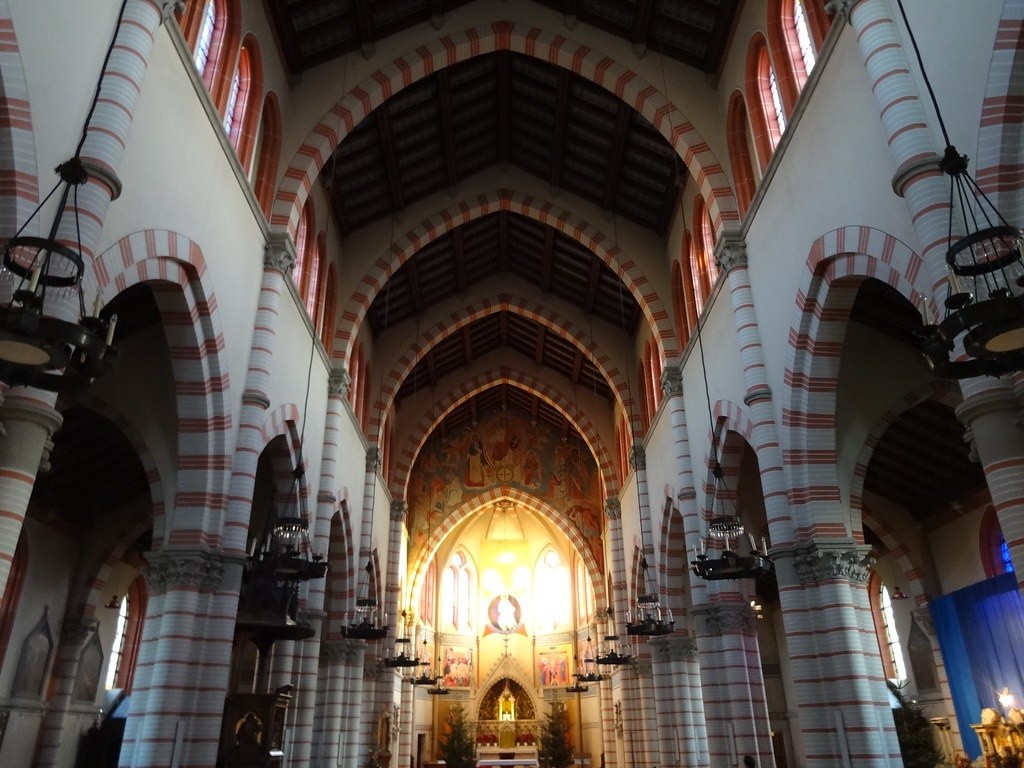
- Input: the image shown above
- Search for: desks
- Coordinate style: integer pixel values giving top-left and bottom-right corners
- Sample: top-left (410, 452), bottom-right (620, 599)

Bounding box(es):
top-left (477, 759), bottom-right (540, 768)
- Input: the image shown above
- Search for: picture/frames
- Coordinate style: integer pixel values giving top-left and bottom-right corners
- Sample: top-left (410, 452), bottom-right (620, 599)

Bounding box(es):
top-left (438, 642), bottom-right (474, 691)
top-left (538, 648), bottom-right (572, 687)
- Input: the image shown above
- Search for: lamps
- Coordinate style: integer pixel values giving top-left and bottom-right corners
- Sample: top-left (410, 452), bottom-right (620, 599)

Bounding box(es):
top-left (247, 0), bottom-right (355, 578)
top-left (384, 288), bottom-right (423, 666)
top-left (654, 0), bottom-right (775, 579)
top-left (339, 208), bottom-right (395, 638)
top-left (426, 418), bottom-right (449, 694)
top-left (562, 414), bottom-right (589, 691)
top-left (612, 200), bottom-right (675, 635)
top-left (409, 336), bottom-right (435, 684)
top-left (1, 0), bottom-right (128, 394)
top-left (587, 286), bottom-right (631, 664)
top-left (897, 2), bottom-right (1024, 376)
top-left (571, 344), bottom-right (603, 681)
